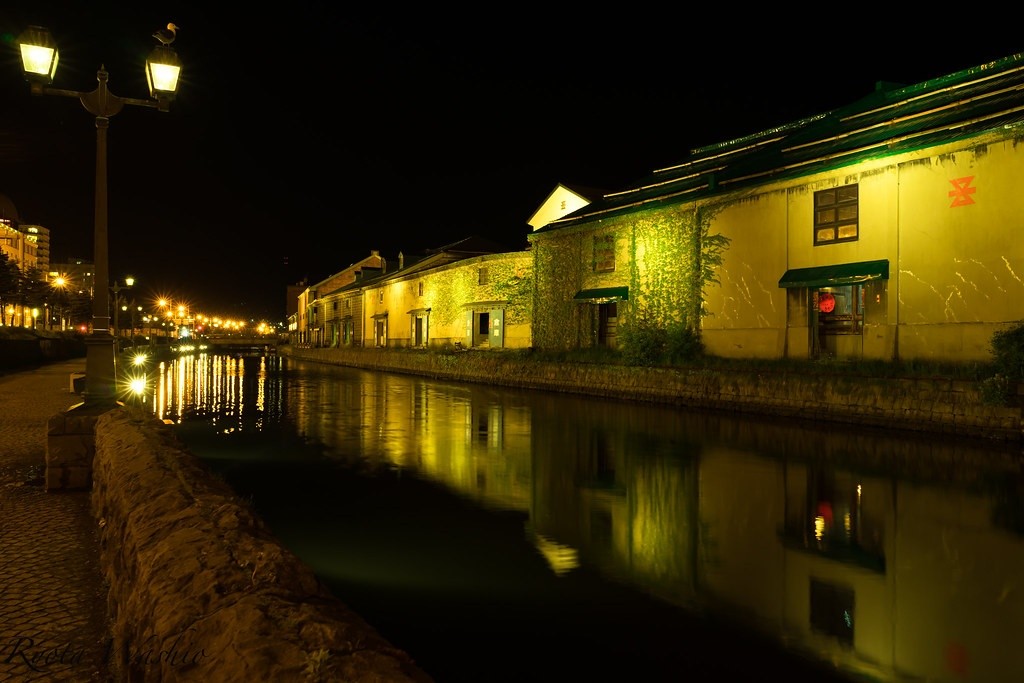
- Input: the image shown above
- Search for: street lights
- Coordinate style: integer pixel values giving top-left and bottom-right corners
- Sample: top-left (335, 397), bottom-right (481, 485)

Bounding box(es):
top-left (122, 305), bottom-right (143, 337)
top-left (142, 315), bottom-right (159, 345)
top-left (111, 278), bottom-right (135, 337)
top-left (19, 23), bottom-right (182, 407)
